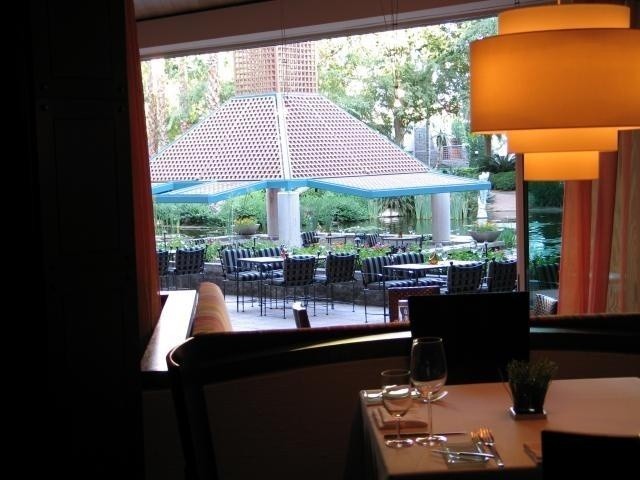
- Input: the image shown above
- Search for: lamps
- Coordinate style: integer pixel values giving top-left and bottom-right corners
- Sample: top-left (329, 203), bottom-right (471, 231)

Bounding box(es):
top-left (469, 1), bottom-right (640, 181)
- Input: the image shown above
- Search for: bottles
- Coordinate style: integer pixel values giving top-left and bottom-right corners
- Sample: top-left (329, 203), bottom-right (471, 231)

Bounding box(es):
top-left (429, 251), bottom-right (438, 265)
top-left (281, 246), bottom-right (288, 258)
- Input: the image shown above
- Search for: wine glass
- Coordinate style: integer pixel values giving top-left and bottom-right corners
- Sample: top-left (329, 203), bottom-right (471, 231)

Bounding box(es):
top-left (435, 242), bottom-right (448, 264)
top-left (381, 336), bottom-right (448, 448)
top-left (287, 248), bottom-right (293, 258)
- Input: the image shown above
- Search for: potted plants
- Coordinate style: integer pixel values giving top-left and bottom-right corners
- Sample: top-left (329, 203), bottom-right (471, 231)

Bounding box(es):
top-left (231, 213), bottom-right (262, 235)
top-left (377, 195), bottom-right (419, 249)
top-left (470, 225), bottom-right (502, 241)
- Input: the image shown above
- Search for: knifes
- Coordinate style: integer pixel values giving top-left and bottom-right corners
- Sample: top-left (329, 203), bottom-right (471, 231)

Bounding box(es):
top-left (430, 449), bottom-right (495, 458)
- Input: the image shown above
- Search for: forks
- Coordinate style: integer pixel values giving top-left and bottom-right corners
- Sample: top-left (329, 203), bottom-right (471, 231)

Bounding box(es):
top-left (471, 425), bottom-right (503, 466)
